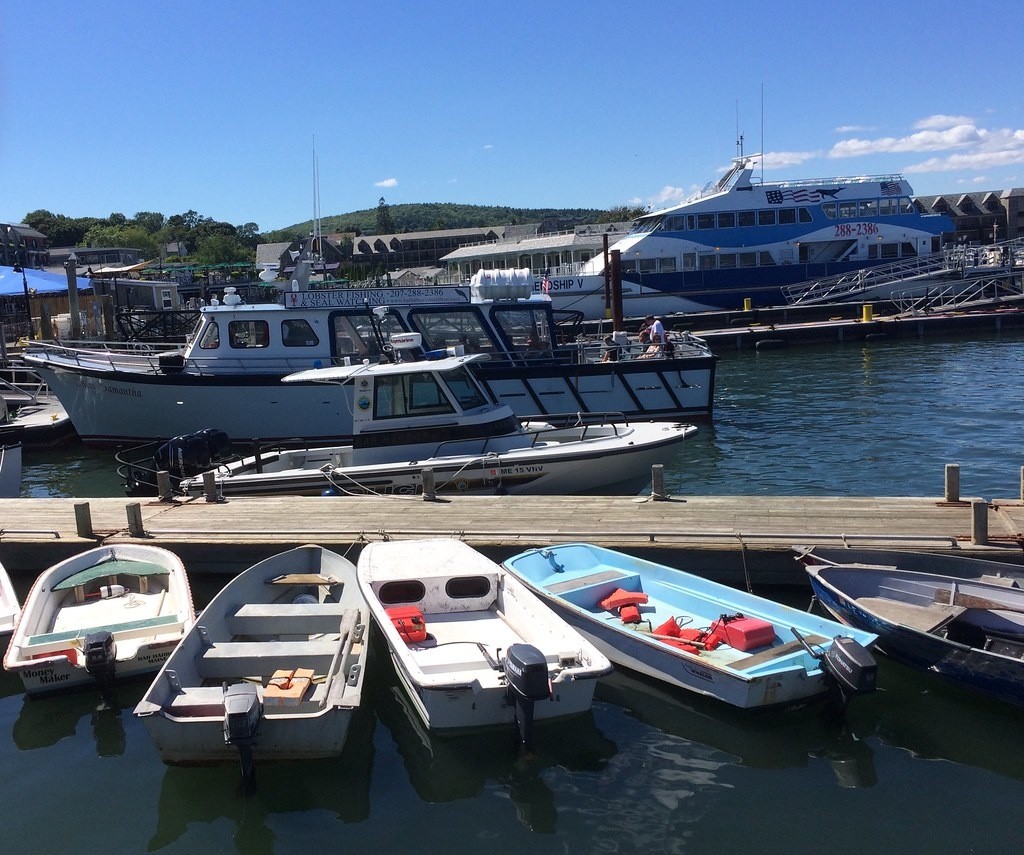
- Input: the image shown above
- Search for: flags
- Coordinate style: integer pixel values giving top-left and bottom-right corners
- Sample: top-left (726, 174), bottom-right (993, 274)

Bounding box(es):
top-left (543, 266), bottom-right (550, 299)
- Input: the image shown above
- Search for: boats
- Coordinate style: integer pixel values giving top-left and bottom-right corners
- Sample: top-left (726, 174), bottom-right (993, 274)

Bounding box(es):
top-left (17, 130), bottom-right (721, 453)
top-left (0, 441), bottom-right (25, 500)
top-left (112, 299), bottom-right (702, 506)
top-left (503, 542), bottom-right (884, 713)
top-left (802, 561), bottom-right (1024, 700)
top-left (786, 541), bottom-right (1024, 606)
top-left (0, 554), bottom-right (26, 637)
top-left (133, 543), bottom-right (377, 767)
top-left (355, 537), bottom-right (615, 734)
top-left (484, 82), bottom-right (1012, 323)
top-left (2, 544), bottom-right (194, 697)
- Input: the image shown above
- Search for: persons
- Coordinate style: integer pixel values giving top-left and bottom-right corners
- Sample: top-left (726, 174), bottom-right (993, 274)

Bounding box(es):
top-left (600, 314), bottom-right (665, 361)
